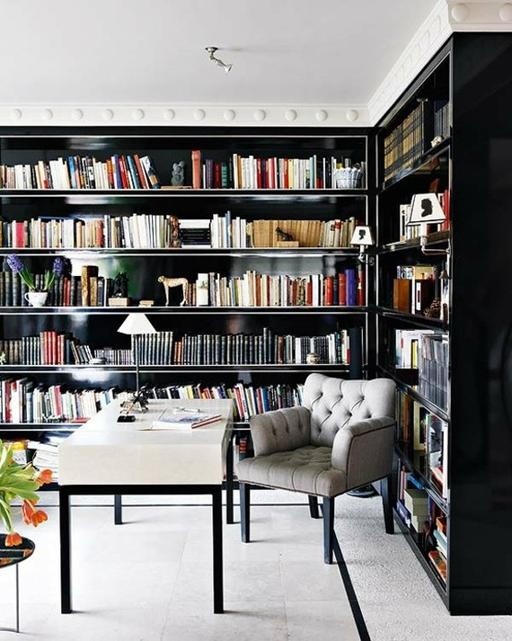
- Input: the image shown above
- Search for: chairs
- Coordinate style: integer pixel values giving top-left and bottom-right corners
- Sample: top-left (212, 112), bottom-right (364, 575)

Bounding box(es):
top-left (234, 372), bottom-right (400, 565)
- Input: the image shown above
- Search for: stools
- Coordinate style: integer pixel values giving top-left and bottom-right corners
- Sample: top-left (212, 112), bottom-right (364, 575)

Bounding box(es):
top-left (0, 534), bottom-right (35, 634)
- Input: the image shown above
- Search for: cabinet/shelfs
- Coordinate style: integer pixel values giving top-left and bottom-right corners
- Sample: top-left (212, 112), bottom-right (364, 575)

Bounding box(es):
top-left (0, 125), bottom-right (373, 433)
top-left (369, 30), bottom-right (512, 618)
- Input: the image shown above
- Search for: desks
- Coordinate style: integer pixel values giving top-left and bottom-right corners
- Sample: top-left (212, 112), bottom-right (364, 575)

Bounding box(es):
top-left (56, 398), bottom-right (236, 616)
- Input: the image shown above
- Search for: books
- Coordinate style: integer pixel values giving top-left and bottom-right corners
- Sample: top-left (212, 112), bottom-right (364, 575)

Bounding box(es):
top-left (383, 99), bottom-right (449, 182)
top-left (0, 376), bottom-right (119, 424)
top-left (0, 152), bottom-right (161, 190)
top-left (397, 466), bottom-right (447, 590)
top-left (210, 210), bottom-right (359, 249)
top-left (398, 189), bottom-right (449, 242)
top-left (394, 387), bottom-right (448, 500)
top-left (0, 265), bottom-right (113, 309)
top-left (393, 327), bottom-right (449, 413)
top-left (191, 148), bottom-right (365, 190)
top-left (0, 213), bottom-right (171, 248)
top-left (186, 264), bottom-right (364, 306)
top-left (152, 410), bottom-right (223, 429)
top-left (391, 252), bottom-right (450, 323)
top-left (141, 383), bottom-right (305, 421)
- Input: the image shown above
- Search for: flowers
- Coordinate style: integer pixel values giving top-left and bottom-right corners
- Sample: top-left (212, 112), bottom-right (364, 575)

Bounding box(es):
top-left (0, 438), bottom-right (51, 549)
top-left (5, 254), bottom-right (63, 291)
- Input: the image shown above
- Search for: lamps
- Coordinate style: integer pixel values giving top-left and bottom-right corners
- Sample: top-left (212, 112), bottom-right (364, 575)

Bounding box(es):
top-left (405, 191), bottom-right (452, 258)
top-left (349, 226), bottom-right (376, 267)
top-left (116, 312), bottom-right (158, 397)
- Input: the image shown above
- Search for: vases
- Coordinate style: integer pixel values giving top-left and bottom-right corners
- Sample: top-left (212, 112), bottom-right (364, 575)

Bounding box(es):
top-left (332, 167), bottom-right (361, 189)
